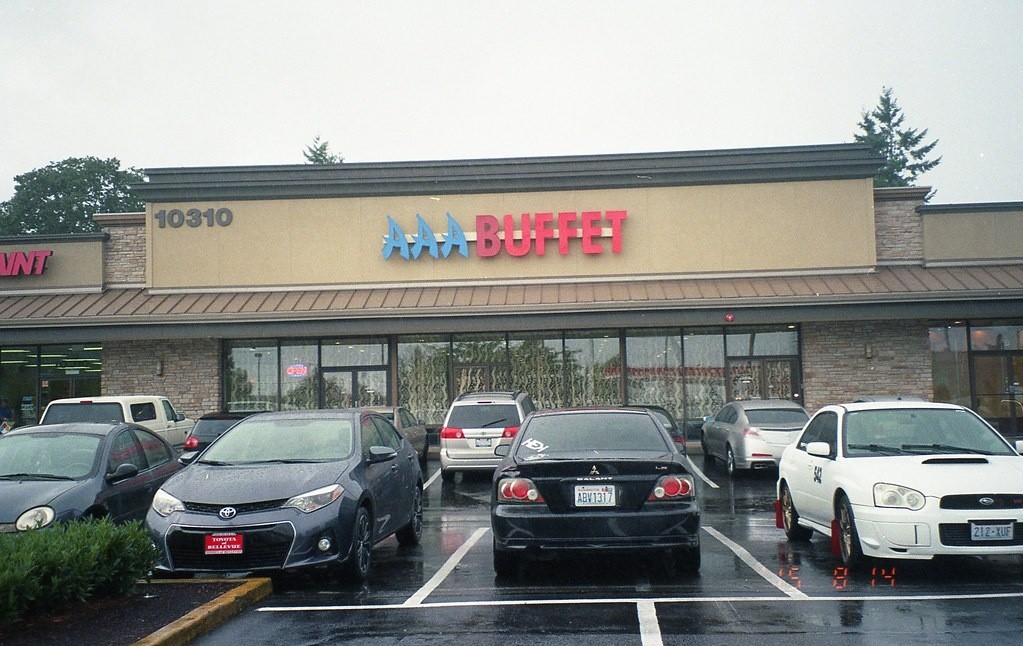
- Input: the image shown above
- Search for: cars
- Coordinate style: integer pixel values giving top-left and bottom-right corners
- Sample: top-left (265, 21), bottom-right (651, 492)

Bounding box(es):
top-left (699, 399), bottom-right (811, 475)
top-left (490, 409), bottom-right (700, 575)
top-left (619, 404), bottom-right (685, 457)
top-left (143, 408), bottom-right (423, 580)
top-left (776, 401), bottom-right (1022, 570)
top-left (363, 407), bottom-right (428, 464)
top-left (39, 395), bottom-right (196, 450)
top-left (0, 422), bottom-right (182, 531)
top-left (180, 410), bottom-right (270, 462)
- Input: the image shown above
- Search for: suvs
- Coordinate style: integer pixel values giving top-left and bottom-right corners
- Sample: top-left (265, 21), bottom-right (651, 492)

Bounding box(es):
top-left (440, 391), bottom-right (537, 481)
